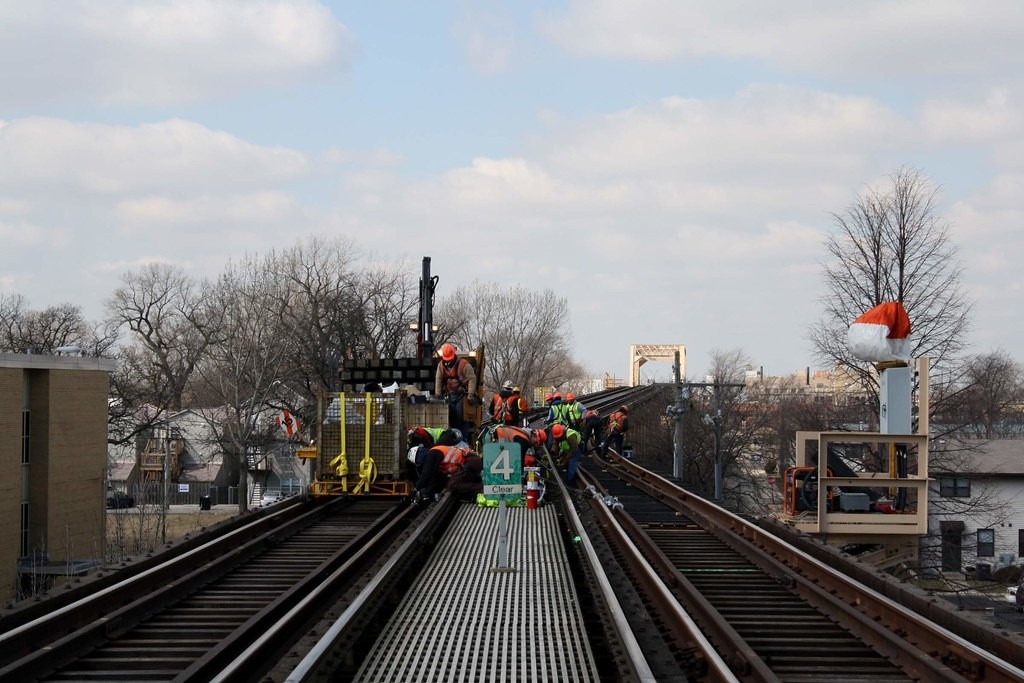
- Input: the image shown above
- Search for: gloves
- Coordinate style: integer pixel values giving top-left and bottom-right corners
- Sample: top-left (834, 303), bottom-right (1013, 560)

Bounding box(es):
top-left (409, 487), bottom-right (417, 499)
top-left (467, 394), bottom-right (475, 406)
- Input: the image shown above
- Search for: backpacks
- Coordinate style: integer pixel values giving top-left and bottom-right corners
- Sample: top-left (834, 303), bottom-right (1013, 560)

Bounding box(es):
top-left (607, 413), bottom-right (625, 436)
top-left (585, 409), bottom-right (598, 418)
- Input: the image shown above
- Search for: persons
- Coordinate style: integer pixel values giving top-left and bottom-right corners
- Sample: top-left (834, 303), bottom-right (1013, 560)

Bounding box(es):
top-left (404, 343), bottom-right (631, 504)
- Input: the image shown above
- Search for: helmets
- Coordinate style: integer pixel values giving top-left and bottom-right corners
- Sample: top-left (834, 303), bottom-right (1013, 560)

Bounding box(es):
top-left (451, 429), bottom-right (462, 445)
top-left (503, 380), bottom-right (513, 388)
top-left (513, 387), bottom-right (521, 391)
top-left (554, 393), bottom-right (561, 398)
top-left (552, 424), bottom-right (563, 438)
top-left (442, 344), bottom-right (455, 360)
top-left (566, 394), bottom-right (575, 400)
top-left (545, 394), bottom-right (553, 401)
top-left (535, 429), bottom-right (546, 446)
top-left (408, 443), bottom-right (424, 466)
top-left (621, 406), bottom-right (628, 413)
